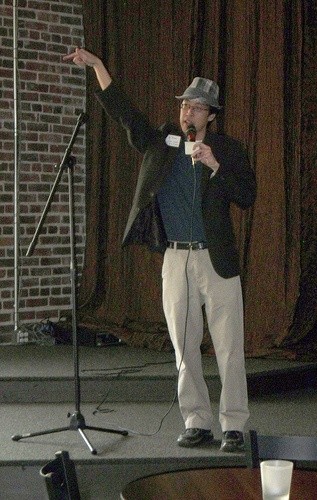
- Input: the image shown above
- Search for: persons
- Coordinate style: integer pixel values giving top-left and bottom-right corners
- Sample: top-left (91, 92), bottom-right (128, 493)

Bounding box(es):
top-left (63, 47), bottom-right (258, 451)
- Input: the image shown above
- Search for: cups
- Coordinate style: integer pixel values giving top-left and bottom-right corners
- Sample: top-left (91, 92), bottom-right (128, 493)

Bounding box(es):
top-left (260, 460), bottom-right (293, 500)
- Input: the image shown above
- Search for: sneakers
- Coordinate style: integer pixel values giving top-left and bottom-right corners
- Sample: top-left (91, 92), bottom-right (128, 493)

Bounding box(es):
top-left (177, 428), bottom-right (213, 447)
top-left (221, 430), bottom-right (243, 453)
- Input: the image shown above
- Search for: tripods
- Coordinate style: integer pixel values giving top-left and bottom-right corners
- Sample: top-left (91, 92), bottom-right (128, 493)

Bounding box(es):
top-left (10, 118), bottom-right (128, 454)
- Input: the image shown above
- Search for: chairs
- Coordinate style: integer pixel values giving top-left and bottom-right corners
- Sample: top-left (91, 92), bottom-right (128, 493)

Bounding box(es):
top-left (244, 429), bottom-right (317, 469)
top-left (40, 449), bottom-right (82, 500)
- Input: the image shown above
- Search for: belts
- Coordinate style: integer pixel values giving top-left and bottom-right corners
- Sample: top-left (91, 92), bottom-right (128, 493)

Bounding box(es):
top-left (166, 241), bottom-right (207, 250)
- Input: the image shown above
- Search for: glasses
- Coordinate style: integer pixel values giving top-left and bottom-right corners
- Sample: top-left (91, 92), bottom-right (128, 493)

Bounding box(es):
top-left (180, 103), bottom-right (211, 113)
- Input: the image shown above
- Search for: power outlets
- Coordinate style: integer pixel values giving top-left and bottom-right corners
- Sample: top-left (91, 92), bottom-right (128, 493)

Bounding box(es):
top-left (16, 329), bottom-right (29, 343)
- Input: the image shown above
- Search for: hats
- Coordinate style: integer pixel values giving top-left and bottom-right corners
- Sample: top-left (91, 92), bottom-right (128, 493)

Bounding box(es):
top-left (174, 77), bottom-right (223, 110)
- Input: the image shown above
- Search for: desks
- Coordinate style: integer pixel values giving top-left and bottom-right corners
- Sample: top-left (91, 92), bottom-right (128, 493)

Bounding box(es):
top-left (120, 465), bottom-right (317, 500)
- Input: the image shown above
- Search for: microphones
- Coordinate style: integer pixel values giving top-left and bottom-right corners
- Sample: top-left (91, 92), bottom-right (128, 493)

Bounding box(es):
top-left (185, 126), bottom-right (199, 168)
